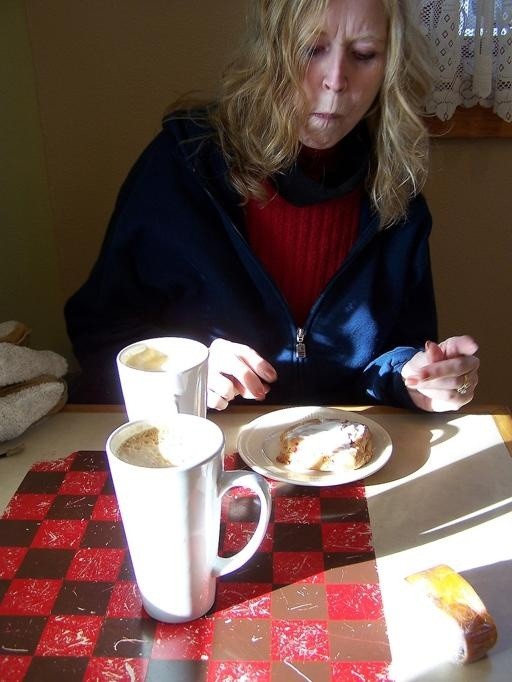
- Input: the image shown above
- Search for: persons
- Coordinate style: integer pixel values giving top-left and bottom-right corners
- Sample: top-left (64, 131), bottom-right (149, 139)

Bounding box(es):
top-left (63, 1), bottom-right (482, 415)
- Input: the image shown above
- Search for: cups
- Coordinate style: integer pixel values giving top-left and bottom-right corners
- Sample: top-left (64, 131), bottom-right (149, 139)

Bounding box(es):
top-left (107, 412), bottom-right (274, 623)
top-left (115, 338), bottom-right (211, 421)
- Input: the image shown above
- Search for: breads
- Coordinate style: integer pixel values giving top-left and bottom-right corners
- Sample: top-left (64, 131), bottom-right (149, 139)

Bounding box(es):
top-left (276, 417), bottom-right (376, 473)
top-left (403, 564), bottom-right (498, 665)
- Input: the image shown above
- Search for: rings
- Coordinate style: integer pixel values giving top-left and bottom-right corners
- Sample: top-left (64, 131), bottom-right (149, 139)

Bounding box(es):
top-left (458, 375), bottom-right (472, 395)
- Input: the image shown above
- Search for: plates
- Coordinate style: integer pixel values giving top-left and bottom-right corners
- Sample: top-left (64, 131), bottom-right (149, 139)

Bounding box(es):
top-left (237, 404), bottom-right (395, 486)
